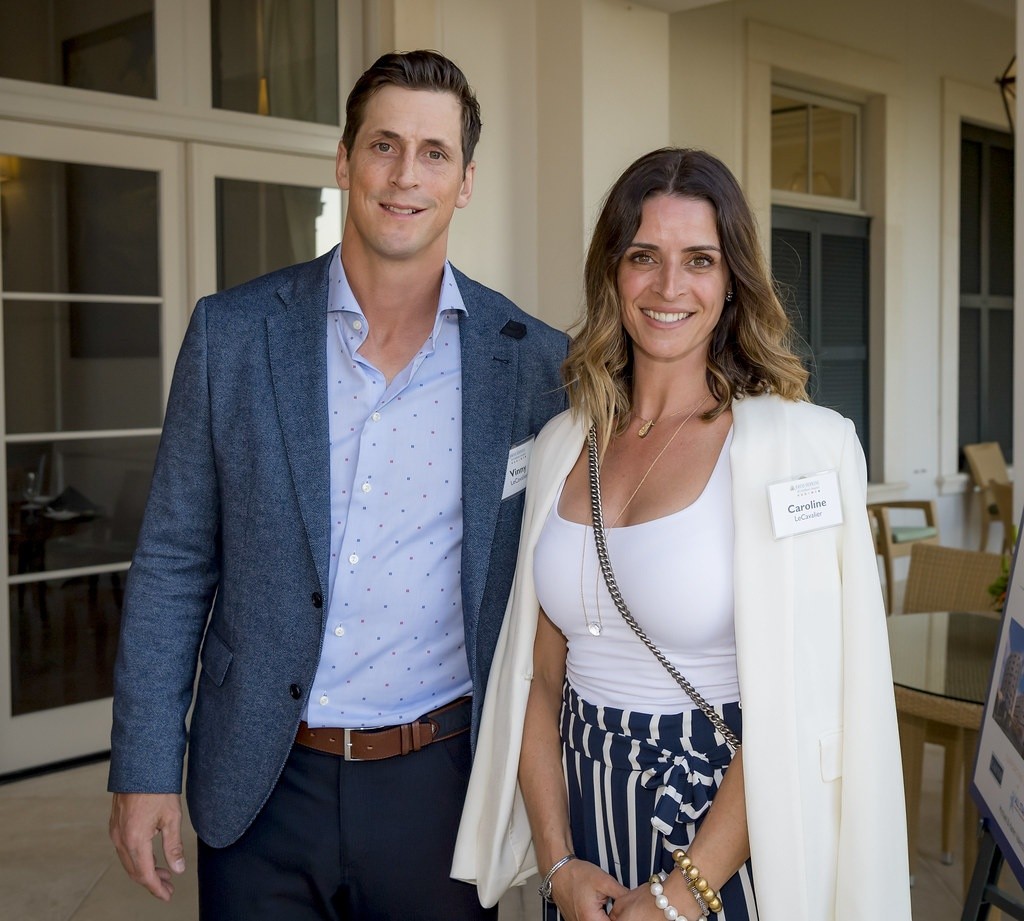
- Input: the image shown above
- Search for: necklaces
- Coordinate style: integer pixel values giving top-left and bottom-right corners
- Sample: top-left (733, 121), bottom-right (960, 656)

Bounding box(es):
top-left (630, 391), bottom-right (711, 439)
top-left (580, 391), bottom-right (711, 637)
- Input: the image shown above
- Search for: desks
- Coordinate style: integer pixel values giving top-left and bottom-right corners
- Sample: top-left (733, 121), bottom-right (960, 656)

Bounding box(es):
top-left (886, 609), bottom-right (1005, 921)
top-left (19, 492), bottom-right (104, 616)
top-left (44, 458), bottom-right (157, 626)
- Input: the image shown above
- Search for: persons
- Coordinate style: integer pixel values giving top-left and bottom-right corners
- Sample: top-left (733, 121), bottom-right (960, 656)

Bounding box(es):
top-left (107, 49), bottom-right (596, 921)
top-left (449, 148), bottom-right (913, 921)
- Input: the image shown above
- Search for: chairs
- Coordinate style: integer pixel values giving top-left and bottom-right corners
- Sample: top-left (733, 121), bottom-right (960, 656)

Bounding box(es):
top-left (987, 477), bottom-right (1013, 556)
top-left (902, 543), bottom-right (1014, 865)
top-left (865, 500), bottom-right (940, 617)
top-left (963, 441), bottom-right (1011, 552)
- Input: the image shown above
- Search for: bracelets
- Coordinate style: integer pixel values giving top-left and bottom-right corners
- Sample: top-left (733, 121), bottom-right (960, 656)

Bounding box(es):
top-left (536, 854), bottom-right (577, 903)
top-left (649, 848), bottom-right (722, 921)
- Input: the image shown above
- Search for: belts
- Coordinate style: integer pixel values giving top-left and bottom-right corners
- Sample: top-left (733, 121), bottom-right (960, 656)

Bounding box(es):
top-left (295, 697), bottom-right (471, 761)
